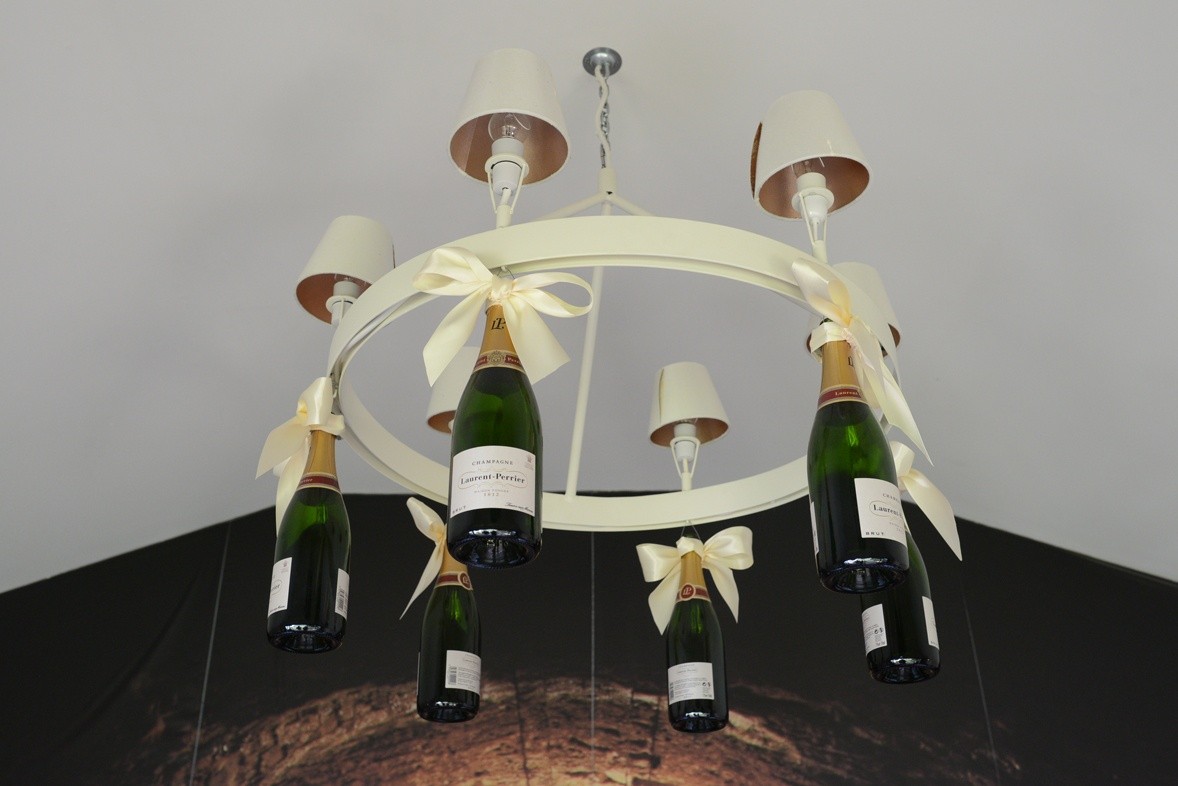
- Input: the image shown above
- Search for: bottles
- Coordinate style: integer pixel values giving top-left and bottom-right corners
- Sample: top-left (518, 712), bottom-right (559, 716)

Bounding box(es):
top-left (808, 318), bottom-right (912, 596)
top-left (447, 304), bottom-right (542, 567)
top-left (860, 505), bottom-right (941, 684)
top-left (665, 538), bottom-right (729, 733)
top-left (265, 430), bottom-right (351, 653)
top-left (416, 544), bottom-right (482, 722)
